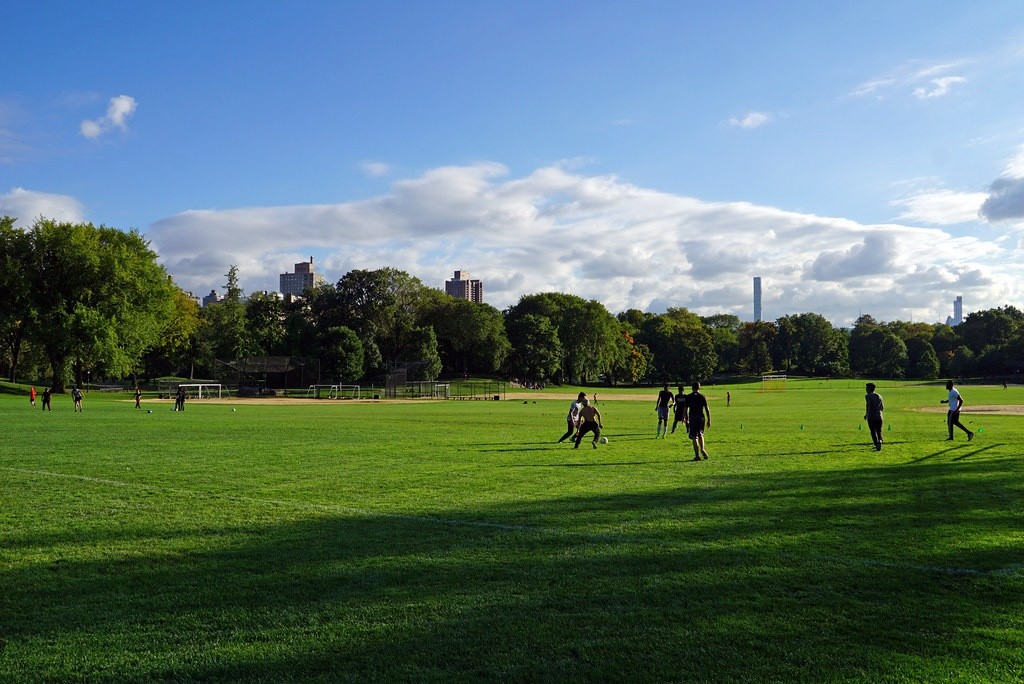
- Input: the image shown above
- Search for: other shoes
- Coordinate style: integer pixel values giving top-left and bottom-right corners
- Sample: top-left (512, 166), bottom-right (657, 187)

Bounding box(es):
top-left (876, 440), bottom-right (882, 451)
top-left (968, 432), bottom-right (974, 441)
top-left (945, 438), bottom-right (954, 441)
top-left (694, 457), bottom-right (701, 460)
top-left (592, 441), bottom-right (597, 449)
top-left (701, 450), bottom-right (708, 459)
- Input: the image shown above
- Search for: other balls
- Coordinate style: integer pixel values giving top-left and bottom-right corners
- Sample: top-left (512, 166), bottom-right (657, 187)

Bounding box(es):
top-left (599, 437), bottom-right (609, 445)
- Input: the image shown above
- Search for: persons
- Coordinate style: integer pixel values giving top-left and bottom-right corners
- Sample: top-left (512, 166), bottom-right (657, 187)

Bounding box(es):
top-left (557, 392), bottom-right (587, 443)
top-left (133, 388), bottom-right (142, 410)
top-left (727, 392), bottom-right (731, 407)
top-left (671, 386), bottom-right (690, 434)
top-left (655, 384), bottom-right (675, 440)
top-left (593, 392), bottom-right (599, 405)
top-left (41, 388), bottom-right (52, 412)
top-left (682, 382), bottom-right (711, 461)
top-left (864, 383), bottom-right (885, 452)
top-left (173, 387), bottom-right (186, 412)
top-left (1002, 381), bottom-right (1009, 390)
top-left (574, 399), bottom-right (603, 449)
top-left (941, 379), bottom-right (974, 442)
top-left (28, 386), bottom-right (37, 408)
top-left (71, 385), bottom-right (85, 412)
